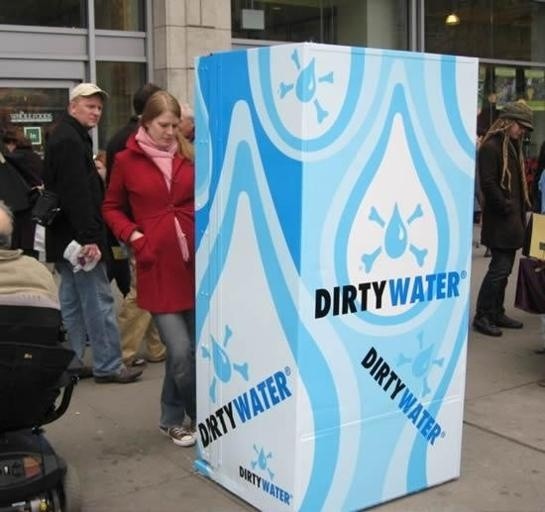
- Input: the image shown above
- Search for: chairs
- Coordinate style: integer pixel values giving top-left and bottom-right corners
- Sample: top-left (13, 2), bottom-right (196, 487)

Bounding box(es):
top-left (2, 342), bottom-right (82, 433)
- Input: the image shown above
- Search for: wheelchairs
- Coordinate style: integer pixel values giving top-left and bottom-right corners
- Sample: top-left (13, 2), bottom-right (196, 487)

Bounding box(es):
top-left (0, 309), bottom-right (83, 512)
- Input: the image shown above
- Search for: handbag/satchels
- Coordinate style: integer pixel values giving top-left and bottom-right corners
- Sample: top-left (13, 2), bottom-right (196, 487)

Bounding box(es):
top-left (32, 191), bottom-right (64, 227)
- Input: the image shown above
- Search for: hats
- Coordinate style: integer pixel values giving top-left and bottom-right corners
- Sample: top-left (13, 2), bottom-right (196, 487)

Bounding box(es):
top-left (69, 83), bottom-right (109, 102)
top-left (499, 102), bottom-right (534, 132)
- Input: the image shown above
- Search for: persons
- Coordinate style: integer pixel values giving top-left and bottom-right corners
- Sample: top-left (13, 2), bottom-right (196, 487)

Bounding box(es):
top-left (469, 95), bottom-right (535, 337)
top-left (176, 108), bottom-right (195, 142)
top-left (101, 82), bottom-right (170, 370)
top-left (43, 82), bottom-right (143, 387)
top-left (1, 108), bottom-right (129, 259)
top-left (475, 89), bottom-right (502, 227)
top-left (0, 199), bottom-right (60, 309)
top-left (97, 88), bottom-right (198, 447)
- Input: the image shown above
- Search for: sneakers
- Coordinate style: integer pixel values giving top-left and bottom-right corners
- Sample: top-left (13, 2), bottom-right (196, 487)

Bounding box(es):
top-left (190, 418), bottom-right (198, 437)
top-left (94, 365), bottom-right (143, 383)
top-left (473, 313), bottom-right (502, 336)
top-left (130, 352), bottom-right (167, 367)
top-left (160, 424), bottom-right (195, 446)
top-left (491, 313), bottom-right (522, 328)
top-left (70, 364), bottom-right (92, 379)
